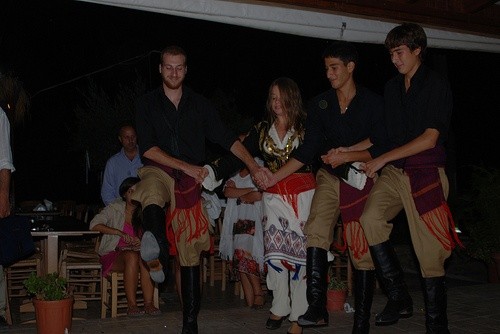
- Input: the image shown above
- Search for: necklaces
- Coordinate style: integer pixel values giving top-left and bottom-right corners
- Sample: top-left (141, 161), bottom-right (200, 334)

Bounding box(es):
top-left (265, 128), bottom-right (299, 160)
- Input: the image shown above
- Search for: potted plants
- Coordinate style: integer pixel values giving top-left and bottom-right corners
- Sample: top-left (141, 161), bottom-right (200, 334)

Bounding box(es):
top-left (22, 270), bottom-right (73, 334)
top-left (454, 171), bottom-right (500, 283)
top-left (327, 270), bottom-right (349, 312)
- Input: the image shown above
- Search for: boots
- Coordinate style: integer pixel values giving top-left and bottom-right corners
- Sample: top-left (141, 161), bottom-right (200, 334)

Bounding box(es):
top-left (180, 265), bottom-right (201, 334)
top-left (368, 239), bottom-right (414, 326)
top-left (140, 203), bottom-right (166, 283)
top-left (297, 247), bottom-right (329, 327)
top-left (352, 270), bottom-right (376, 334)
top-left (420, 274), bottom-right (449, 334)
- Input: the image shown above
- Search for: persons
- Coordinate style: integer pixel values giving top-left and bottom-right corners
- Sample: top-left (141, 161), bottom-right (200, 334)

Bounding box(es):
top-left (0, 106), bottom-right (19, 334)
top-left (195, 76), bottom-right (322, 334)
top-left (328, 22), bottom-right (463, 334)
top-left (89, 177), bottom-right (162, 317)
top-left (253, 41), bottom-right (385, 334)
top-left (218, 166), bottom-right (268, 310)
top-left (100, 124), bottom-right (146, 205)
top-left (127, 46), bottom-right (268, 334)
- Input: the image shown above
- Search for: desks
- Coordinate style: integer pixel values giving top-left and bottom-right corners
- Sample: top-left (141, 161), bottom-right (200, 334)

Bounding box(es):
top-left (31, 231), bottom-right (100, 312)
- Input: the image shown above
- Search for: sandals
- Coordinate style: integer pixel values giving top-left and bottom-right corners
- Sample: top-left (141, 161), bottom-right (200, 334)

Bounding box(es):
top-left (127, 304), bottom-right (146, 316)
top-left (145, 304), bottom-right (162, 315)
top-left (251, 294), bottom-right (264, 310)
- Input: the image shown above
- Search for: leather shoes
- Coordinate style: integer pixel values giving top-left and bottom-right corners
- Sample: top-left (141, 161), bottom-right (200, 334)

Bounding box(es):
top-left (287, 326), bottom-right (303, 334)
top-left (266, 313), bottom-right (290, 330)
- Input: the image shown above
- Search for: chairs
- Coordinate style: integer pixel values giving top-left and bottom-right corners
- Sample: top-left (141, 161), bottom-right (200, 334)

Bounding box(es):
top-left (198, 207), bottom-right (245, 300)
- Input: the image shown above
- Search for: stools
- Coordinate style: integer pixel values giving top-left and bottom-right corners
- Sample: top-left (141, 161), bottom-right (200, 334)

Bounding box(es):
top-left (0, 249), bottom-right (159, 326)
